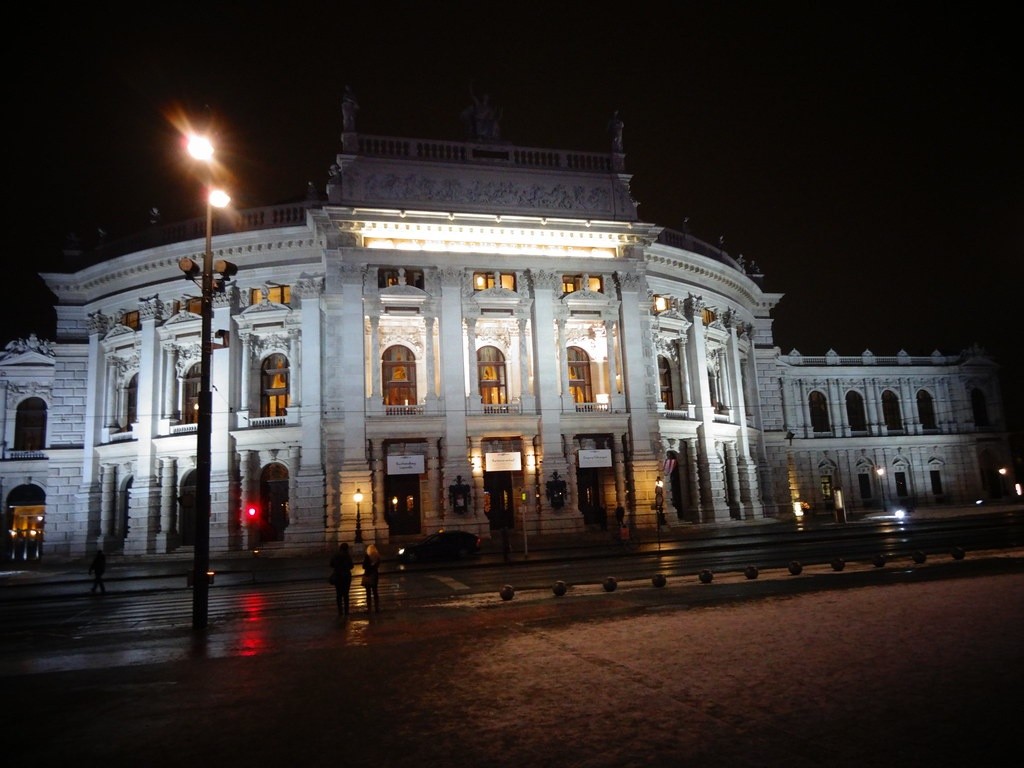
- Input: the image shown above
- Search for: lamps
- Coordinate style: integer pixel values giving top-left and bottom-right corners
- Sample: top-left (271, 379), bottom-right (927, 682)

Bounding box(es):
top-left (653, 294), bottom-right (665, 312)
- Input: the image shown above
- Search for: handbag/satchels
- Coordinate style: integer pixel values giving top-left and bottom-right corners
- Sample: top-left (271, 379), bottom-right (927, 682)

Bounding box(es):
top-left (330, 573), bottom-right (336, 585)
top-left (362, 572), bottom-right (378, 587)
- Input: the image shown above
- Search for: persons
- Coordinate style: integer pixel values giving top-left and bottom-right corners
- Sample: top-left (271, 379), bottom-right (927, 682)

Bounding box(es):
top-left (615, 503), bottom-right (624, 526)
top-left (364, 545), bottom-right (383, 614)
top-left (329, 543), bottom-right (353, 619)
top-left (89, 549), bottom-right (106, 592)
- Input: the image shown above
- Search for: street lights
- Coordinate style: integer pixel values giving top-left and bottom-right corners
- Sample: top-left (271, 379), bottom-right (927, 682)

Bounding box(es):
top-left (353, 488), bottom-right (364, 544)
top-left (177, 130), bottom-right (240, 649)
top-left (877, 468), bottom-right (888, 512)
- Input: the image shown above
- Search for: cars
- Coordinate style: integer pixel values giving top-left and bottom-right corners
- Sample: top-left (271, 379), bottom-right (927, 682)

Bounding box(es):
top-left (395, 529), bottom-right (481, 564)
